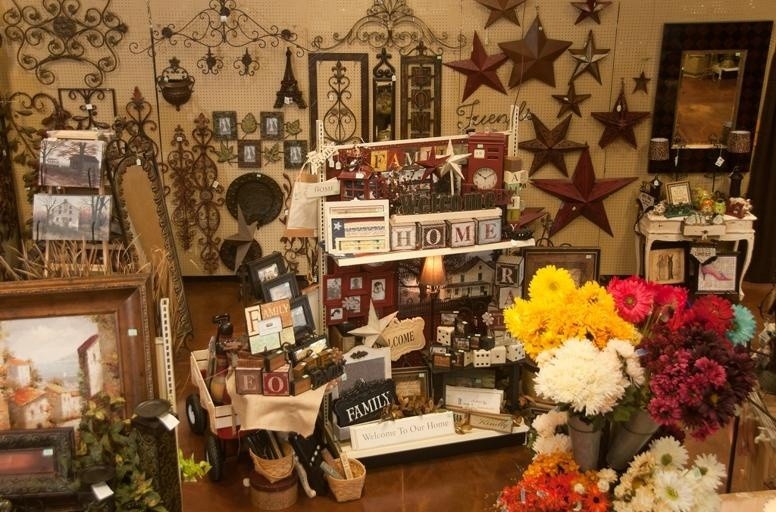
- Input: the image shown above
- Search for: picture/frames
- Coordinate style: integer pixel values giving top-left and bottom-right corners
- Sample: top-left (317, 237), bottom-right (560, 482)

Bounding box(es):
top-left (322, 258), bottom-right (393, 325)
top-left (391, 366), bottom-right (435, 411)
top-left (208, 106), bottom-right (237, 139)
top-left (664, 182), bottom-right (695, 205)
top-left (695, 252), bottom-right (739, 294)
top-left (251, 250), bottom-right (315, 345)
top-left (652, 240), bottom-right (689, 284)
top-left (286, 142), bottom-right (311, 167)
top-left (0, 276), bottom-right (162, 511)
top-left (235, 140), bottom-right (263, 172)
top-left (258, 108), bottom-right (288, 136)
top-left (519, 246), bottom-right (604, 301)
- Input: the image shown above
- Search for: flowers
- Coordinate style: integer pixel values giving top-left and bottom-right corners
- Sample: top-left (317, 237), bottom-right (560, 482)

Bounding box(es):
top-left (483, 261), bottom-right (761, 511)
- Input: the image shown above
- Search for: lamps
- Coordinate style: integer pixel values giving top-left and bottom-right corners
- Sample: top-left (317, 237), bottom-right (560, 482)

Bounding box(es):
top-left (729, 131), bottom-right (752, 199)
top-left (646, 131), bottom-right (667, 203)
top-left (722, 119), bottom-right (729, 142)
top-left (419, 258), bottom-right (450, 338)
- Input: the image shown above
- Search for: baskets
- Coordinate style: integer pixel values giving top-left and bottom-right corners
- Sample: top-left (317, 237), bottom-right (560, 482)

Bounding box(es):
top-left (325, 458), bottom-right (366, 502)
top-left (248, 438), bottom-right (295, 483)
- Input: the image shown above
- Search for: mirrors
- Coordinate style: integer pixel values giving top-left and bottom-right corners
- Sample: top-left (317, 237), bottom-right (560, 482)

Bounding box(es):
top-left (647, 20), bottom-right (771, 174)
top-left (105, 147), bottom-right (193, 347)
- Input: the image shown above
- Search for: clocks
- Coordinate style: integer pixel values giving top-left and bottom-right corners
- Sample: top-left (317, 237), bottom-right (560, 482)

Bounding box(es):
top-left (461, 131), bottom-right (506, 195)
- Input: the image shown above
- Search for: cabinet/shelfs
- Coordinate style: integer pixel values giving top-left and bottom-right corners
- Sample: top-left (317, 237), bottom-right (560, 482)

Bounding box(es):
top-left (308, 103), bottom-right (536, 462)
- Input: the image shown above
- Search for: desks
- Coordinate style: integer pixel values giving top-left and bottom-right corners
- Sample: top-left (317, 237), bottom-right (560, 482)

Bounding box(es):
top-left (633, 209), bottom-right (757, 301)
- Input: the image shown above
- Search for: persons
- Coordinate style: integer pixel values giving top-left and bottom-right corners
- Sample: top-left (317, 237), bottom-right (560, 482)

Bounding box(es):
top-left (666, 251), bottom-right (674, 279)
top-left (373, 282), bottom-right (385, 299)
top-left (290, 147), bottom-right (302, 163)
top-left (352, 279), bottom-right (360, 290)
top-left (266, 118), bottom-right (278, 134)
top-left (220, 119), bottom-right (231, 134)
top-left (331, 309), bottom-right (341, 319)
top-left (244, 146), bottom-right (255, 162)
